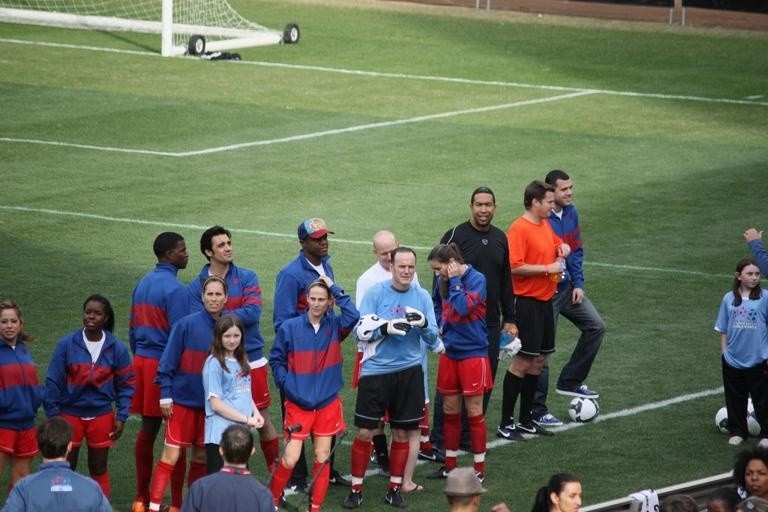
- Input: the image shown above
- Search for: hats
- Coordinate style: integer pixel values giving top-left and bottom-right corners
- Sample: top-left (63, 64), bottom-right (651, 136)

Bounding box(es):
top-left (442, 467), bottom-right (489, 498)
top-left (297, 217), bottom-right (336, 242)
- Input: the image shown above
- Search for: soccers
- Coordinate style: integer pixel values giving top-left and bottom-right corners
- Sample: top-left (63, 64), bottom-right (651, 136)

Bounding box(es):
top-left (747, 397), bottom-right (761, 436)
top-left (715, 406), bottom-right (730, 434)
top-left (568, 397), bottom-right (599, 423)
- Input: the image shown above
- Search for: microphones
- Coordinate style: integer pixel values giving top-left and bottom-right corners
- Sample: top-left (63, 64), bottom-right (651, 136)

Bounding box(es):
top-left (287, 424), bottom-right (302, 432)
top-left (341, 430), bottom-right (349, 438)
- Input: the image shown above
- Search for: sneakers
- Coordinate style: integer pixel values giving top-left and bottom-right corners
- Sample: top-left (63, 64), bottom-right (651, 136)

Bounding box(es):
top-left (446, 447), bottom-right (470, 458)
top-left (417, 448), bottom-right (446, 464)
top-left (534, 412), bottom-right (564, 427)
top-left (476, 469), bottom-right (486, 485)
top-left (342, 484), bottom-right (409, 509)
top-left (516, 422), bottom-right (554, 438)
top-left (728, 435), bottom-right (744, 446)
top-left (496, 424), bottom-right (528, 443)
top-left (458, 440), bottom-right (488, 455)
top-left (757, 437), bottom-right (767, 450)
top-left (427, 465), bottom-right (449, 480)
top-left (555, 382), bottom-right (600, 399)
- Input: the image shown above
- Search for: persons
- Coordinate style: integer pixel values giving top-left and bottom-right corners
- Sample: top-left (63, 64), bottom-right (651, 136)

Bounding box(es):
top-left (742, 225), bottom-right (768, 281)
top-left (126, 225), bottom-right (275, 511)
top-left (531, 166), bottom-right (607, 426)
top-left (0, 415), bottom-right (116, 512)
top-left (714, 259), bottom-right (768, 446)
top-left (426, 186), bottom-right (517, 485)
top-left (344, 230), bottom-right (445, 507)
top-left (494, 175), bottom-right (573, 442)
top-left (268, 217), bottom-right (362, 512)
top-left (0, 298), bottom-right (42, 499)
top-left (658, 444), bottom-right (768, 512)
top-left (42, 294), bottom-right (136, 499)
top-left (531, 472), bottom-right (583, 512)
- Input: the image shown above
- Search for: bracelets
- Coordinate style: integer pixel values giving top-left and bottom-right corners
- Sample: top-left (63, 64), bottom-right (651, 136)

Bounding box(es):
top-left (543, 264), bottom-right (548, 276)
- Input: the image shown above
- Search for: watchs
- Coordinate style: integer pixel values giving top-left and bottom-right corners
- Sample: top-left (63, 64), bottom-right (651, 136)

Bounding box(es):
top-left (447, 285), bottom-right (465, 291)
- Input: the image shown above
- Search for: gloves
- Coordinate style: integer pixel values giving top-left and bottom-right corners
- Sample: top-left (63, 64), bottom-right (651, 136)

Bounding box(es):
top-left (405, 304), bottom-right (429, 330)
top-left (381, 316), bottom-right (409, 337)
